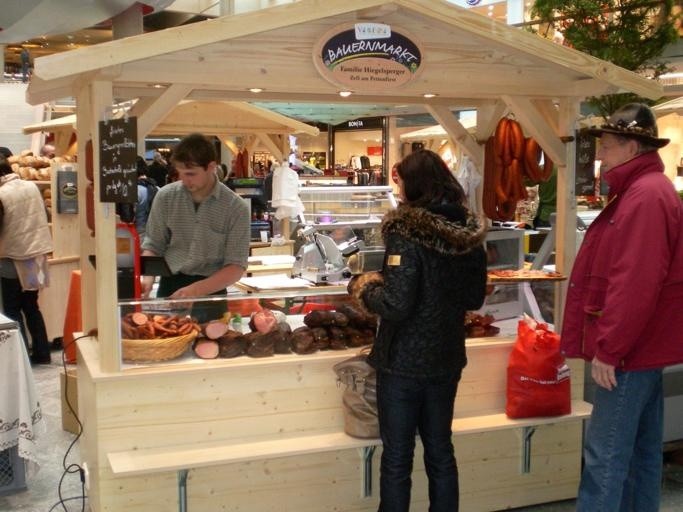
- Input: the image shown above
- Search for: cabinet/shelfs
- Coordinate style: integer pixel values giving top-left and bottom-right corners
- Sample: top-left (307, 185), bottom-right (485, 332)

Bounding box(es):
top-left (73, 266), bottom-right (596, 511)
top-left (1, 154), bottom-right (647, 350)
top-left (1, 314), bottom-right (48, 497)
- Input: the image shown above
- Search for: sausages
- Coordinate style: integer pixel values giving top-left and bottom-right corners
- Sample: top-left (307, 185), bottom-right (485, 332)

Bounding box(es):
top-left (121, 312), bottom-right (202, 339)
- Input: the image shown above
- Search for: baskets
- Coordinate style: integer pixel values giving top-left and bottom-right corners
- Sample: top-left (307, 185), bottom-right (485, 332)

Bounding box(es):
top-left (95, 313), bottom-right (199, 362)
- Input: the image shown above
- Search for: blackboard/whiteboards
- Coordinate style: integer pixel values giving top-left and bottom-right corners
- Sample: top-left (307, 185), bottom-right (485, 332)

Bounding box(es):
top-left (98, 116), bottom-right (138, 202)
top-left (575, 123), bottom-right (596, 197)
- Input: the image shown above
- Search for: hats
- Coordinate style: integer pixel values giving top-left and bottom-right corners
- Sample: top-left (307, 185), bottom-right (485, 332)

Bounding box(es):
top-left (585, 102), bottom-right (670, 148)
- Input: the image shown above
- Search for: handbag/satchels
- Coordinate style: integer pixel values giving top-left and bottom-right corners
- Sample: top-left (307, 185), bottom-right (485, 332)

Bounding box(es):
top-left (332, 354), bottom-right (420, 439)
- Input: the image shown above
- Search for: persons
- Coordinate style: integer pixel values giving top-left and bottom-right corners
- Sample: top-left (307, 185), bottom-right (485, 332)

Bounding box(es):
top-left (264, 158), bottom-right (274, 171)
top-left (560, 101), bottom-right (682, 510)
top-left (0, 145), bottom-right (13, 162)
top-left (0, 154), bottom-right (53, 366)
top-left (347, 150), bottom-right (486, 510)
top-left (136, 133), bottom-right (250, 319)
top-left (131, 148), bottom-right (177, 246)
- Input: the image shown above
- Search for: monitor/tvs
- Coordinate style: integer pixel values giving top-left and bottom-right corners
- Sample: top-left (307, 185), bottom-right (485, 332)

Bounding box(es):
top-left (471, 230), bottom-right (524, 324)
